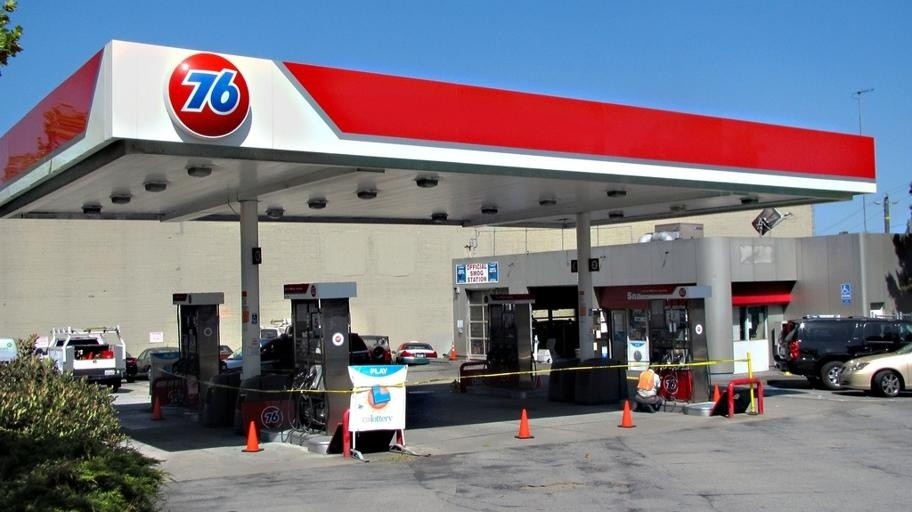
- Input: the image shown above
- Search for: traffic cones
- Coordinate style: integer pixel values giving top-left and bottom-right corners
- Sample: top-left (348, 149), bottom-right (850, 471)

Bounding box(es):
top-left (515, 410), bottom-right (534, 437)
top-left (151, 399), bottom-right (163, 420)
top-left (713, 385), bottom-right (720, 402)
top-left (618, 400), bottom-right (636, 428)
top-left (449, 343), bottom-right (458, 360)
top-left (243, 421), bottom-right (263, 452)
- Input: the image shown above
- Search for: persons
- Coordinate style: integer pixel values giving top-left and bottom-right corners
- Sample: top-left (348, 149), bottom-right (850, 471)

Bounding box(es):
top-left (632, 361), bottom-right (662, 413)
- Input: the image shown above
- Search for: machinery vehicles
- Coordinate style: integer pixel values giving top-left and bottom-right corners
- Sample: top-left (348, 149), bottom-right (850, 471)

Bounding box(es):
top-left (49, 325), bottom-right (126, 392)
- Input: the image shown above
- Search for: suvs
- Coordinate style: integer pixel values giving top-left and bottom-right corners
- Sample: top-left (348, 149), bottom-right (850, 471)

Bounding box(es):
top-left (361, 334), bottom-right (392, 364)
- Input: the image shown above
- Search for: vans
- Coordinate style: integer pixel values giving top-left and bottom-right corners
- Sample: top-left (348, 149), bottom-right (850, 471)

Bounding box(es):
top-left (779, 317), bottom-right (912, 390)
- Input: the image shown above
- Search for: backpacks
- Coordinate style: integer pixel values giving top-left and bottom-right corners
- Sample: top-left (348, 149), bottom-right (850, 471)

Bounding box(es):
top-left (637, 371), bottom-right (655, 391)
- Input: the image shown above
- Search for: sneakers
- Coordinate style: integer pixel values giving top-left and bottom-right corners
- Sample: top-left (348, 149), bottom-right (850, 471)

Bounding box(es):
top-left (647, 404), bottom-right (656, 413)
top-left (634, 402), bottom-right (639, 411)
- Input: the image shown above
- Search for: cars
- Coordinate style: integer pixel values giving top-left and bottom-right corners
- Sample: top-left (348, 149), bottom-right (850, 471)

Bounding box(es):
top-left (221, 327), bottom-right (369, 369)
top-left (126, 346), bottom-right (178, 381)
top-left (840, 342), bottom-right (912, 397)
top-left (394, 342), bottom-right (437, 365)
